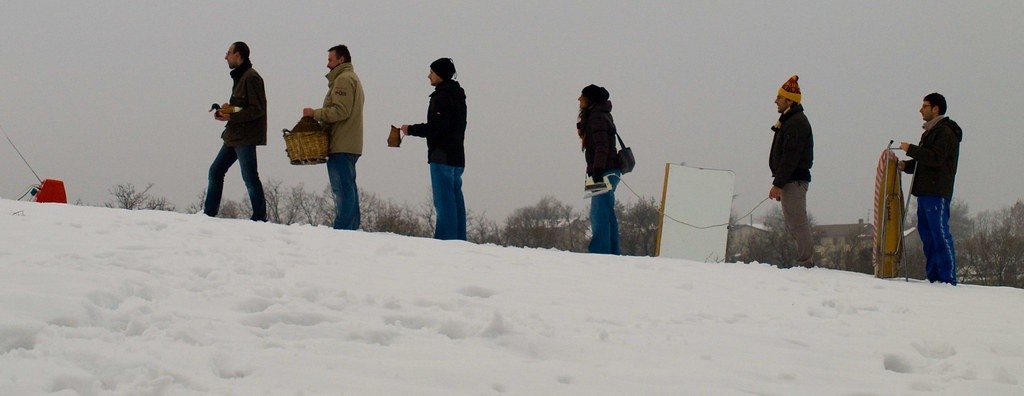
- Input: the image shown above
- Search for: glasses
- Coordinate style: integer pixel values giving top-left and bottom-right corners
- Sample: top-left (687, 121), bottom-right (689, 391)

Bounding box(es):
top-left (922, 103), bottom-right (932, 108)
top-left (226, 50), bottom-right (237, 55)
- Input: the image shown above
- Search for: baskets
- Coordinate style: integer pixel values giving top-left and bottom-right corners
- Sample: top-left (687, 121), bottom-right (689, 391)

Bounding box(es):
top-left (281, 124), bottom-right (332, 164)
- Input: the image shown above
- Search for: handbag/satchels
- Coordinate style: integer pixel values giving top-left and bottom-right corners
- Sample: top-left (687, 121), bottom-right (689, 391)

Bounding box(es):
top-left (618, 147), bottom-right (635, 175)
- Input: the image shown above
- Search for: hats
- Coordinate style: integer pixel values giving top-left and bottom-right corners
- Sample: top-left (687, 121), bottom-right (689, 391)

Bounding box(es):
top-left (581, 84), bottom-right (609, 105)
top-left (778, 74), bottom-right (802, 104)
top-left (430, 57), bottom-right (456, 81)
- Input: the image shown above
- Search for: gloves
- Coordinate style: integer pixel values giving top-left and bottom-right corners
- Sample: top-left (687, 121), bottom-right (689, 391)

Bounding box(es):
top-left (592, 171), bottom-right (604, 183)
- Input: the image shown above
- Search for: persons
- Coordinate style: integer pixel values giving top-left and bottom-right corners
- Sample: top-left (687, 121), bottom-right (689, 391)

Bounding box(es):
top-left (767, 73), bottom-right (816, 270)
top-left (200, 41), bottom-right (268, 223)
top-left (301, 45), bottom-right (365, 231)
top-left (577, 83), bottom-right (622, 255)
top-left (401, 57), bottom-right (468, 243)
top-left (898, 91), bottom-right (964, 286)
